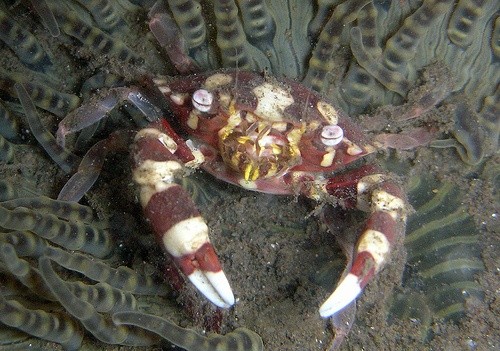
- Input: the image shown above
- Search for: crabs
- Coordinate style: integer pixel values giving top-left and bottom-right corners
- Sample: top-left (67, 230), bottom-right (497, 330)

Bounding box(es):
top-left (57, 1), bottom-right (452, 351)
